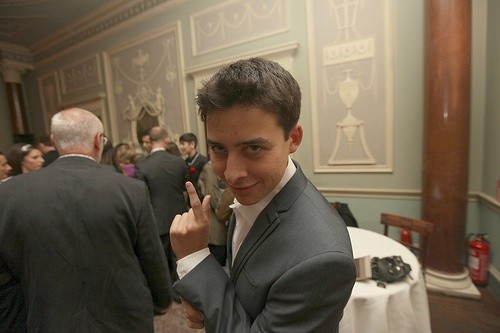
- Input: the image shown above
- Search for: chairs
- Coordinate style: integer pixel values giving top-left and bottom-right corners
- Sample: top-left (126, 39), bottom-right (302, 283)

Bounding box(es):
top-left (380, 213), bottom-right (433, 274)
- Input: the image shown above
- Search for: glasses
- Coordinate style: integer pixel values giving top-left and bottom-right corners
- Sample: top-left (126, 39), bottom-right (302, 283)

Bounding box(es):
top-left (102, 137), bottom-right (107, 144)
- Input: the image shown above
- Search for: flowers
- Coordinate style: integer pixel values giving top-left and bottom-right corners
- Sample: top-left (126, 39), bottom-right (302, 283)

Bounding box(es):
top-left (190, 167), bottom-right (196, 173)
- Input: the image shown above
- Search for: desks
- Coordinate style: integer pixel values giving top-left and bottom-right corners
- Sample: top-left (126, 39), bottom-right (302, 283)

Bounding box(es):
top-left (338, 226), bottom-right (432, 333)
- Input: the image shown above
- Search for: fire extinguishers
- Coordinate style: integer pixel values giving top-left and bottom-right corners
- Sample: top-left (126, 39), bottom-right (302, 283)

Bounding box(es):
top-left (467, 233), bottom-right (491, 287)
top-left (400, 230), bottom-right (412, 248)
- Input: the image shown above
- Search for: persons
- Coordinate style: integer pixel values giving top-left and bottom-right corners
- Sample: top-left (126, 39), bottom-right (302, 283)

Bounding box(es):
top-left (100, 126), bottom-right (235, 304)
top-left (0, 108), bottom-right (170, 333)
top-left (170, 57), bottom-right (355, 333)
top-left (0, 131), bottom-right (60, 181)
